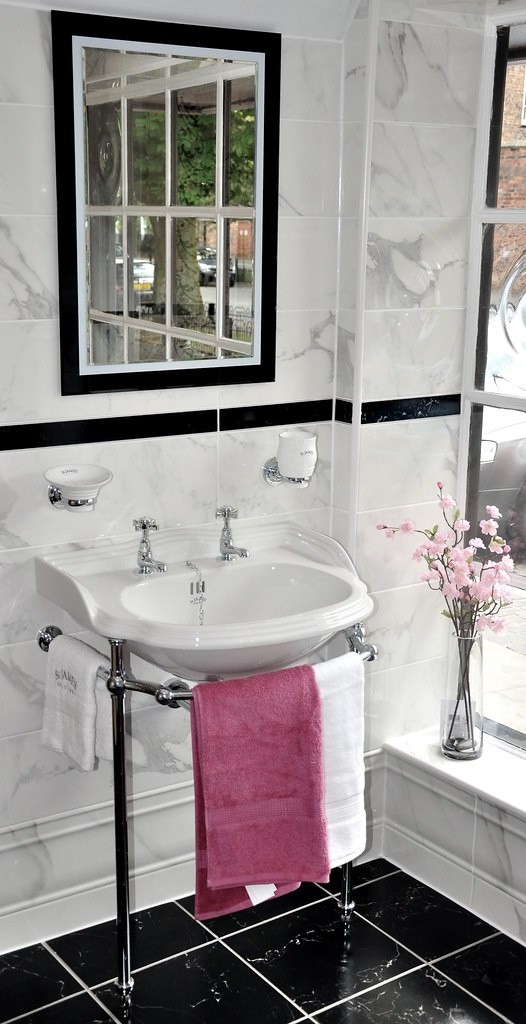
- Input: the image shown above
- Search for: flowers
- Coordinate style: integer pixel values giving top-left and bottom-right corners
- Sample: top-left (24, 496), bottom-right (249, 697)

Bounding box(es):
top-left (376, 482), bottom-right (515, 752)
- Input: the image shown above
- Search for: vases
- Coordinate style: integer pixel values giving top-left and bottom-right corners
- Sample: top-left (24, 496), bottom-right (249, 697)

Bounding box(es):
top-left (440, 630), bottom-right (483, 761)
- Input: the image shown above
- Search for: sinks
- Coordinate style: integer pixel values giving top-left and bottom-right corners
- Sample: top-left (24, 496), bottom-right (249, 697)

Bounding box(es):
top-left (37, 519), bottom-right (379, 688)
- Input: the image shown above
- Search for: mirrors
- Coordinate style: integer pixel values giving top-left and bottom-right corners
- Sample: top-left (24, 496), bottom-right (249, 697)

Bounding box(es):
top-left (50, 10), bottom-right (281, 396)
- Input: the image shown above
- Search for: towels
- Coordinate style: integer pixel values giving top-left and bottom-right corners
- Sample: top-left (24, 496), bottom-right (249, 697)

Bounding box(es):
top-left (189, 663), bottom-right (330, 922)
top-left (312, 650), bottom-right (366, 870)
top-left (39, 634), bottom-right (114, 771)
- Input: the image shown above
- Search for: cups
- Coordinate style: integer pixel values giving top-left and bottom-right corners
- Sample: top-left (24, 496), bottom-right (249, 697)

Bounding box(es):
top-left (278, 431), bottom-right (318, 488)
top-left (44, 464), bottom-right (112, 511)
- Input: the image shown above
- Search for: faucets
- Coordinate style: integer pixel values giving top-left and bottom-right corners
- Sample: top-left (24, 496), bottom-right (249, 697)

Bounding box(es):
top-left (132, 516), bottom-right (169, 574)
top-left (214, 505), bottom-right (249, 562)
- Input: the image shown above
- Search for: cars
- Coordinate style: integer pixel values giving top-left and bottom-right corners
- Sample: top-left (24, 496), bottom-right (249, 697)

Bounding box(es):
top-left (114, 258), bottom-right (155, 306)
top-left (196, 249), bottom-right (236, 287)
